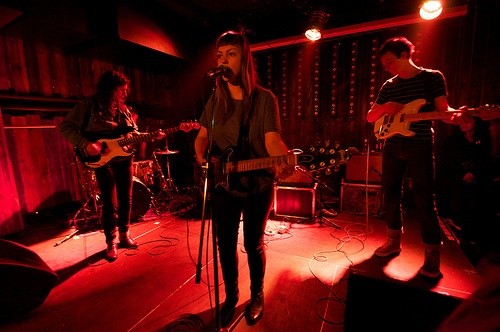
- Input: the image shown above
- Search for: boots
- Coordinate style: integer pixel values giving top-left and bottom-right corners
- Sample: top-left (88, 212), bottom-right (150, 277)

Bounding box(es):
top-left (120, 230), bottom-right (139, 248)
top-left (420, 247), bottom-right (440, 277)
top-left (374, 226), bottom-right (404, 256)
top-left (106, 231), bottom-right (119, 258)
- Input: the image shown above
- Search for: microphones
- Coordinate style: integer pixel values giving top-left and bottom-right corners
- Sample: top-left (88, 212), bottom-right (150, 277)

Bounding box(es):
top-left (205, 64), bottom-right (230, 78)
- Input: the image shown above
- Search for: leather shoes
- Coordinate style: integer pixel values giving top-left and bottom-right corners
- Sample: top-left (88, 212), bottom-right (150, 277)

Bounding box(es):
top-left (219, 297), bottom-right (238, 328)
top-left (250, 292), bottom-right (264, 320)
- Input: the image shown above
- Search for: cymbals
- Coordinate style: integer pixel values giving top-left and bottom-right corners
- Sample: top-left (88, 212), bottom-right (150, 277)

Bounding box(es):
top-left (153, 150), bottom-right (180, 155)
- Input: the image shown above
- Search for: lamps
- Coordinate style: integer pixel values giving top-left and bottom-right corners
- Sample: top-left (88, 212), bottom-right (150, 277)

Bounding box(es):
top-left (305, 11), bottom-right (326, 40)
top-left (419, 0), bottom-right (444, 20)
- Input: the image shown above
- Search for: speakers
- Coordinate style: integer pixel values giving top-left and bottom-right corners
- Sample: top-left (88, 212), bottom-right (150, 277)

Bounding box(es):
top-left (342, 273), bottom-right (463, 332)
top-left (277, 154), bottom-right (316, 186)
top-left (0, 239), bottom-right (60, 315)
top-left (345, 151), bottom-right (383, 185)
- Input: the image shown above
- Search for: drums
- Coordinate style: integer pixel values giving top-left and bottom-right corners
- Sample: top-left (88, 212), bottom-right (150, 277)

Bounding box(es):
top-left (130, 160), bottom-right (155, 187)
top-left (80, 171), bottom-right (99, 186)
top-left (112, 175), bottom-right (152, 224)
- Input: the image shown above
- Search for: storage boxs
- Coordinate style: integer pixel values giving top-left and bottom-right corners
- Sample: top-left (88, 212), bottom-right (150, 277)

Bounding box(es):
top-left (274, 185), bottom-right (321, 220)
top-left (344, 155), bottom-right (384, 182)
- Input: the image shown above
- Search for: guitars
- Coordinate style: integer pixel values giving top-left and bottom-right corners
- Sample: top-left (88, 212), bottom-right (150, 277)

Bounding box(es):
top-left (373, 99), bottom-right (500, 140)
top-left (195, 139), bottom-right (359, 201)
top-left (75, 118), bottom-right (201, 170)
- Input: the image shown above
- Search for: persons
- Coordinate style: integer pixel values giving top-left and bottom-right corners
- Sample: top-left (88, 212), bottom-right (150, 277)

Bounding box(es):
top-left (62, 69), bottom-right (165, 259)
top-left (366, 38), bottom-right (473, 281)
top-left (195, 32), bottom-right (294, 326)
